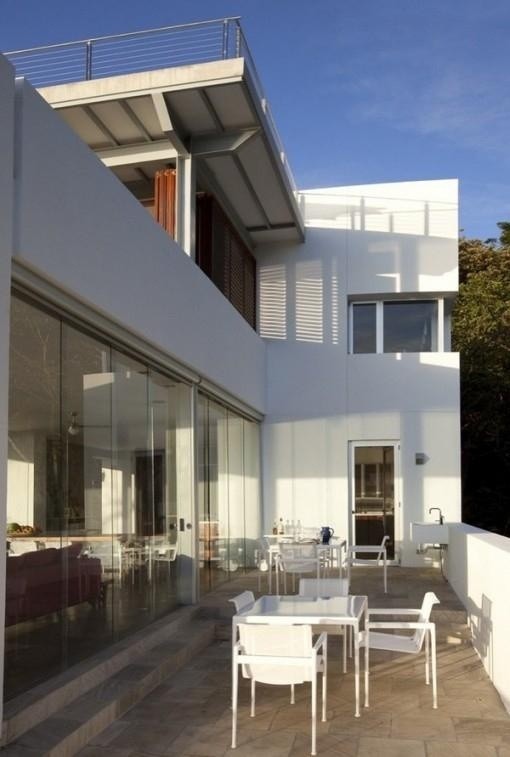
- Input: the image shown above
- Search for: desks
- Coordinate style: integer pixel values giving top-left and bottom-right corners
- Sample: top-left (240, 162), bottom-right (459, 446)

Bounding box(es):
top-left (231, 593), bottom-right (375, 721)
top-left (8, 533), bottom-right (124, 544)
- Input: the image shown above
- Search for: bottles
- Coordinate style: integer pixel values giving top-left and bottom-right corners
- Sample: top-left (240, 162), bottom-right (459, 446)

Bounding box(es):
top-left (271, 517), bottom-right (285, 535)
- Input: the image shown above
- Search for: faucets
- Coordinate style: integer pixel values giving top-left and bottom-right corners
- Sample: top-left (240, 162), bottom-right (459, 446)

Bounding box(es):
top-left (429, 507), bottom-right (444, 525)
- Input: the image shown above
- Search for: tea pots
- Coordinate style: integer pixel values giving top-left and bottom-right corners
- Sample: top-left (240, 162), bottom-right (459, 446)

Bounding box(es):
top-left (320, 526), bottom-right (334, 545)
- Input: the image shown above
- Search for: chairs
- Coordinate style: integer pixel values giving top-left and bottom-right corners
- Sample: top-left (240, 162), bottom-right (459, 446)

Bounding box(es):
top-left (352, 590), bottom-right (438, 713)
top-left (228, 622), bottom-right (332, 757)
top-left (229, 589), bottom-right (256, 610)
top-left (229, 529), bottom-right (397, 600)
top-left (298, 576), bottom-right (352, 673)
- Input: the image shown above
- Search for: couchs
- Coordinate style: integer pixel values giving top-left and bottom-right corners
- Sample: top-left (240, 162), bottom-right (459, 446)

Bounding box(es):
top-left (6, 542), bottom-right (103, 628)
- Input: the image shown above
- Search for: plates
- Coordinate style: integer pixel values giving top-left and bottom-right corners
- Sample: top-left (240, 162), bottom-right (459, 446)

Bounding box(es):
top-left (7, 532), bottom-right (40, 537)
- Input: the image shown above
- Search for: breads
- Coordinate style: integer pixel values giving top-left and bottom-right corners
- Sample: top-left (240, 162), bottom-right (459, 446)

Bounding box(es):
top-left (16, 526), bottom-right (33, 535)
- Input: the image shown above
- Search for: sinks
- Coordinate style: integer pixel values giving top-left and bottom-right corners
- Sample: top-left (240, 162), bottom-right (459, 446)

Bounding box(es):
top-left (410, 520), bottom-right (449, 545)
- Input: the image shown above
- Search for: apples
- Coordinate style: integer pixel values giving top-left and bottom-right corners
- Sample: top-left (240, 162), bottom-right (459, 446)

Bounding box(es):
top-left (7, 523), bottom-right (19, 532)
